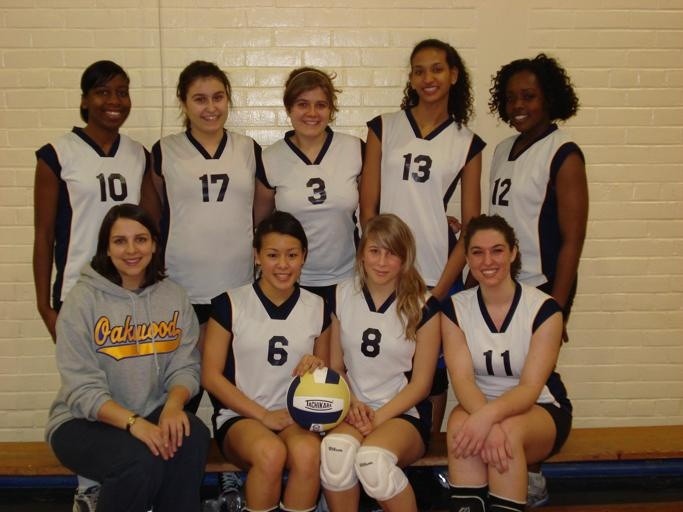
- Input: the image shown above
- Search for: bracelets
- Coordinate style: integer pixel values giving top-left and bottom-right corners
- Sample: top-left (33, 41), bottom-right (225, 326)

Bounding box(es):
top-left (125, 413), bottom-right (141, 434)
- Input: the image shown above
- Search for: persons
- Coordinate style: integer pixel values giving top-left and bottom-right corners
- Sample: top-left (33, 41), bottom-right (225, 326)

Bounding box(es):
top-left (31, 59), bottom-right (169, 511)
top-left (42, 203), bottom-right (211, 511)
top-left (146, 59), bottom-right (260, 511)
top-left (199, 210), bottom-right (332, 511)
top-left (435, 213), bottom-right (574, 512)
top-left (477, 46), bottom-right (588, 510)
top-left (358, 38), bottom-right (487, 511)
top-left (251, 64), bottom-right (365, 510)
top-left (317, 210), bottom-right (443, 510)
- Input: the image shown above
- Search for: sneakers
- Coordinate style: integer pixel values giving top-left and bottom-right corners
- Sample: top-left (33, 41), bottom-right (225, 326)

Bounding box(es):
top-left (526, 471), bottom-right (550, 507)
top-left (71, 486), bottom-right (99, 511)
top-left (213, 470), bottom-right (244, 511)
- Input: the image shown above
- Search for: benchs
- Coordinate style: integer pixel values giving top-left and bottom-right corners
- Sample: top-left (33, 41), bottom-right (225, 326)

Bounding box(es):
top-left (0, 425), bottom-right (683, 490)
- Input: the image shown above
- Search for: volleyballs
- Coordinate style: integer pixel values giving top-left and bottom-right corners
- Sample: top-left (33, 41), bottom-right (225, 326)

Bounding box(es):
top-left (287, 367), bottom-right (351, 433)
